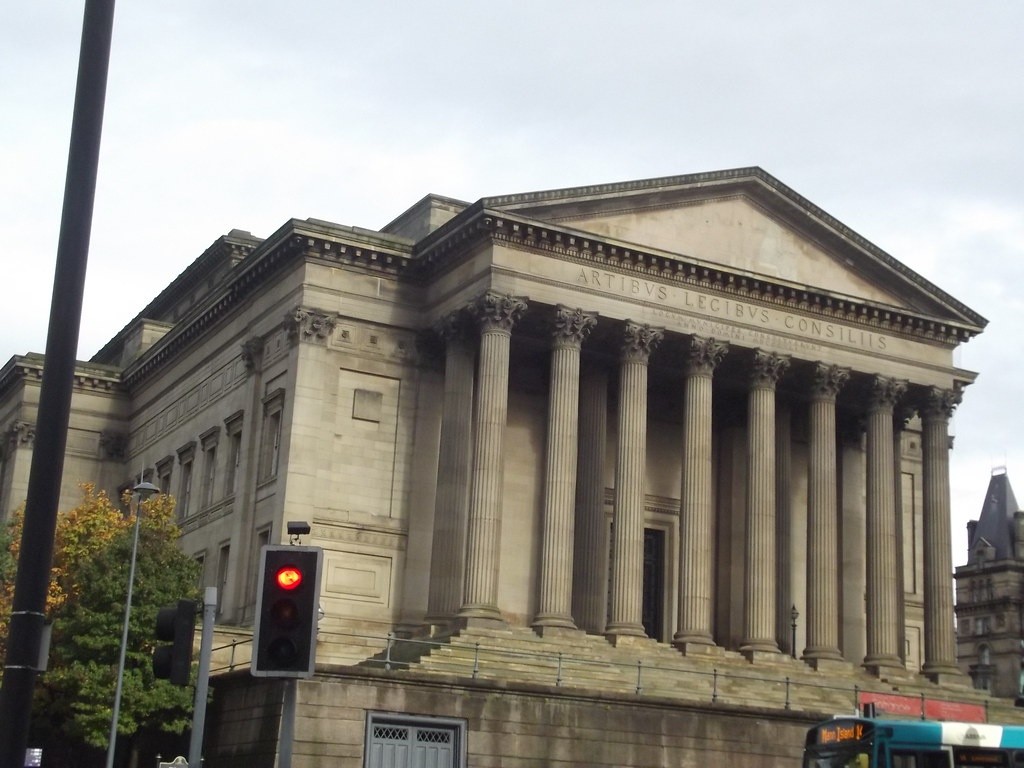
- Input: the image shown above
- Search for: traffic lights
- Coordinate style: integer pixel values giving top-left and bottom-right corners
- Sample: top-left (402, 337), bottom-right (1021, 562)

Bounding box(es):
top-left (153, 597), bottom-right (197, 689)
top-left (251, 544), bottom-right (324, 679)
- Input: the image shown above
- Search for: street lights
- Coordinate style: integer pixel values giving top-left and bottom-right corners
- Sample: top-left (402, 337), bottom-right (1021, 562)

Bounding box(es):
top-left (105, 481), bottom-right (161, 768)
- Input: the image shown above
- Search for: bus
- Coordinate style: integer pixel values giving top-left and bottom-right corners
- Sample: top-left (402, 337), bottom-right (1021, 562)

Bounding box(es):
top-left (801, 713), bottom-right (1024, 768)
